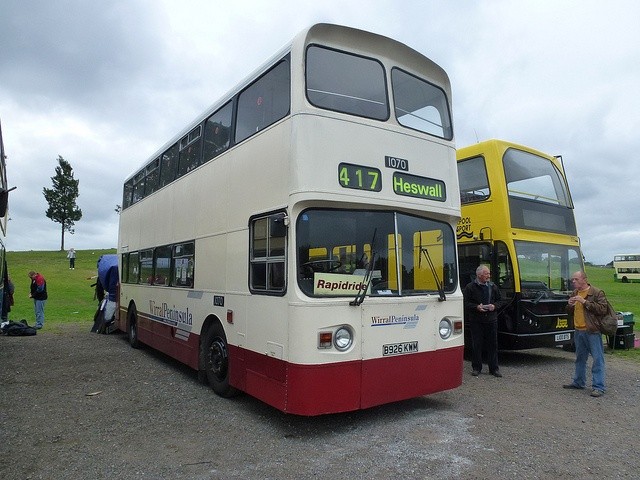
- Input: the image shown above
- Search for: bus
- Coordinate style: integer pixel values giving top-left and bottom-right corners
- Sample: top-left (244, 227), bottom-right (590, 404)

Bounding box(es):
top-left (613, 253), bottom-right (640, 283)
top-left (1, 121), bottom-right (18, 328)
top-left (309, 137), bottom-right (589, 355)
top-left (115, 22), bottom-right (468, 417)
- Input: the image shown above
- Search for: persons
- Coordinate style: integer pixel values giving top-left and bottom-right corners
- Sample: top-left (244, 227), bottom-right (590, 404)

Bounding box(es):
top-left (28, 271), bottom-right (48, 329)
top-left (2, 273), bottom-right (14, 324)
top-left (563, 271), bottom-right (608, 397)
top-left (147, 270), bottom-right (194, 287)
top-left (67, 248), bottom-right (76, 270)
top-left (463, 265), bottom-right (503, 377)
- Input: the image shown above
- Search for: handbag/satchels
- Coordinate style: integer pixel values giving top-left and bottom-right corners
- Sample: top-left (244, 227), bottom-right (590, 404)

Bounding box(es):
top-left (600, 315), bottom-right (617, 335)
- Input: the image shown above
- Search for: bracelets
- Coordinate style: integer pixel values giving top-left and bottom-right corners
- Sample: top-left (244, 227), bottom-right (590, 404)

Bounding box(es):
top-left (583, 300), bottom-right (586, 304)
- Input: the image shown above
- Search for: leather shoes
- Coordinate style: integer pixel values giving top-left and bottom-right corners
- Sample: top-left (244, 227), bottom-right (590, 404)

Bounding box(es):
top-left (589, 391), bottom-right (603, 397)
top-left (489, 370), bottom-right (502, 378)
top-left (471, 370), bottom-right (481, 376)
top-left (563, 384), bottom-right (583, 389)
